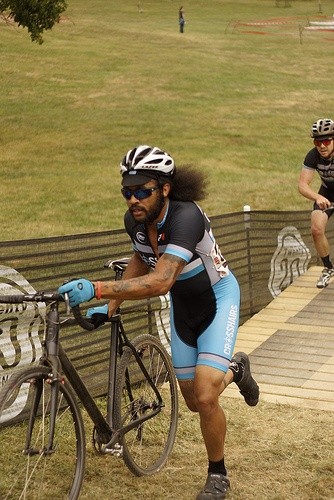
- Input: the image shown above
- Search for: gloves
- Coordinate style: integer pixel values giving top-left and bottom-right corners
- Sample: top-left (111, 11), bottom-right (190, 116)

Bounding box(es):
top-left (59, 279), bottom-right (96, 308)
top-left (87, 303), bottom-right (111, 328)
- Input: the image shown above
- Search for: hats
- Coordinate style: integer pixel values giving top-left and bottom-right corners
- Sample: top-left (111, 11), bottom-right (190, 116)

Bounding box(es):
top-left (120, 175), bottom-right (152, 186)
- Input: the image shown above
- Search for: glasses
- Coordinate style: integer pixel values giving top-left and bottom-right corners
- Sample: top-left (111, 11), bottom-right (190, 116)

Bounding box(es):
top-left (314, 138), bottom-right (333, 146)
top-left (121, 184), bottom-right (161, 200)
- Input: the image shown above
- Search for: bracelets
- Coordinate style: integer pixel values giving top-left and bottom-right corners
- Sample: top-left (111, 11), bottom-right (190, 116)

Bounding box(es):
top-left (96, 281), bottom-right (102, 300)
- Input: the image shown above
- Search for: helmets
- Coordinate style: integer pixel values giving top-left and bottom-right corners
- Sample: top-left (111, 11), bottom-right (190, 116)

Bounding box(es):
top-left (119, 145), bottom-right (176, 185)
top-left (310, 119), bottom-right (334, 137)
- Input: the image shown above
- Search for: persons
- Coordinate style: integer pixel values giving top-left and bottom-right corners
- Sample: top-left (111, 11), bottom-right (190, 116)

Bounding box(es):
top-left (179, 6), bottom-right (186, 33)
top-left (58, 145), bottom-right (259, 500)
top-left (298, 118), bottom-right (334, 289)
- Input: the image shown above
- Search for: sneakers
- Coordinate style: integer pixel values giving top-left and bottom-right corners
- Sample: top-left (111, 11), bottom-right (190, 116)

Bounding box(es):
top-left (196, 472), bottom-right (230, 500)
top-left (317, 267), bottom-right (334, 288)
top-left (231, 352), bottom-right (259, 406)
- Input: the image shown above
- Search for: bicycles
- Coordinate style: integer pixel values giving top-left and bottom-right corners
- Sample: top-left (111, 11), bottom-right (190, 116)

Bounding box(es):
top-left (0, 257), bottom-right (179, 500)
